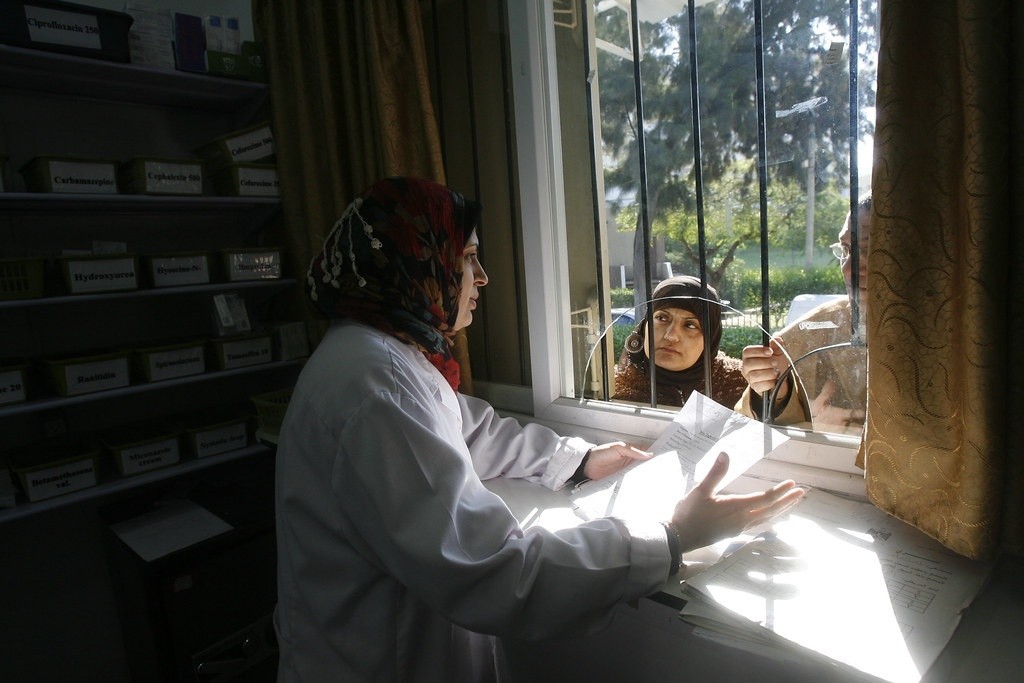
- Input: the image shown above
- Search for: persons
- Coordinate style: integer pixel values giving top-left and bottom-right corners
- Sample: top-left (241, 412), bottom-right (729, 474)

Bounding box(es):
top-left (274, 175), bottom-right (806, 683)
top-left (614, 275), bottom-right (749, 411)
top-left (741, 189), bottom-right (873, 427)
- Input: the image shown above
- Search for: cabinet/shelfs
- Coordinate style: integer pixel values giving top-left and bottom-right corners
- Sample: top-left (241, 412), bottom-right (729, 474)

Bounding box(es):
top-left (0, 43), bottom-right (314, 525)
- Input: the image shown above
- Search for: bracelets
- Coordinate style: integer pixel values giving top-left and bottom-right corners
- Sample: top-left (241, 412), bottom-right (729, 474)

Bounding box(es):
top-left (659, 520), bottom-right (684, 582)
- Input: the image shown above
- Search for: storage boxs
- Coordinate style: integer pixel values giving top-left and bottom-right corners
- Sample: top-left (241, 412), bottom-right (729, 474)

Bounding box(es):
top-left (19, 156), bottom-right (119, 197)
top-left (0, 256), bottom-right (47, 301)
top-left (129, 339), bottom-right (206, 383)
top-left (257, 274), bottom-right (264, 277)
top-left (220, 246), bottom-right (281, 283)
top-left (0, 366), bottom-right (27, 407)
top-left (120, 157), bottom-right (206, 195)
top-left (16, 434), bottom-right (100, 504)
top-left (54, 251), bottom-right (140, 296)
top-left (28, 351), bottom-right (129, 397)
top-left (0, 0), bottom-right (270, 82)
top-left (186, 419), bottom-right (245, 460)
top-left (207, 162), bottom-right (281, 197)
top-left (252, 388), bottom-right (298, 437)
top-left (145, 250), bottom-right (213, 288)
top-left (206, 331), bottom-right (277, 371)
top-left (188, 120), bottom-right (277, 171)
top-left (108, 429), bottom-right (179, 477)
top-left (271, 321), bottom-right (310, 360)
